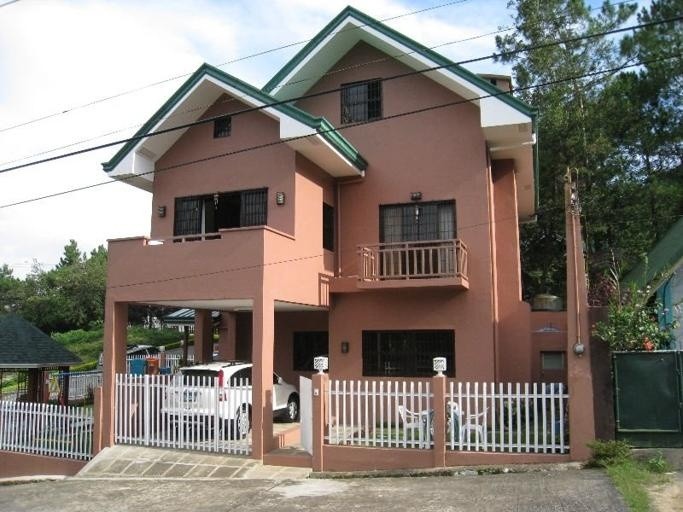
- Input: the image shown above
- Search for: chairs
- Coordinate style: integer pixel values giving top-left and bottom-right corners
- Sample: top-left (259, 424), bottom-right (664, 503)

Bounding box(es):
top-left (460, 406), bottom-right (490, 450)
top-left (397, 404), bottom-right (433, 446)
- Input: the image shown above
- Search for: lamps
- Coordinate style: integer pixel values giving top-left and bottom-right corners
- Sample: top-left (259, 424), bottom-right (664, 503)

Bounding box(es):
top-left (276, 191), bottom-right (286, 206)
top-left (410, 191), bottom-right (424, 200)
top-left (340, 342), bottom-right (349, 352)
top-left (157, 204), bottom-right (166, 217)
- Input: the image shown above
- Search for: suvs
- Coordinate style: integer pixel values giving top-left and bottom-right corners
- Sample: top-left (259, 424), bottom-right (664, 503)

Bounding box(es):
top-left (97, 345), bottom-right (171, 371)
top-left (160, 359), bottom-right (300, 438)
